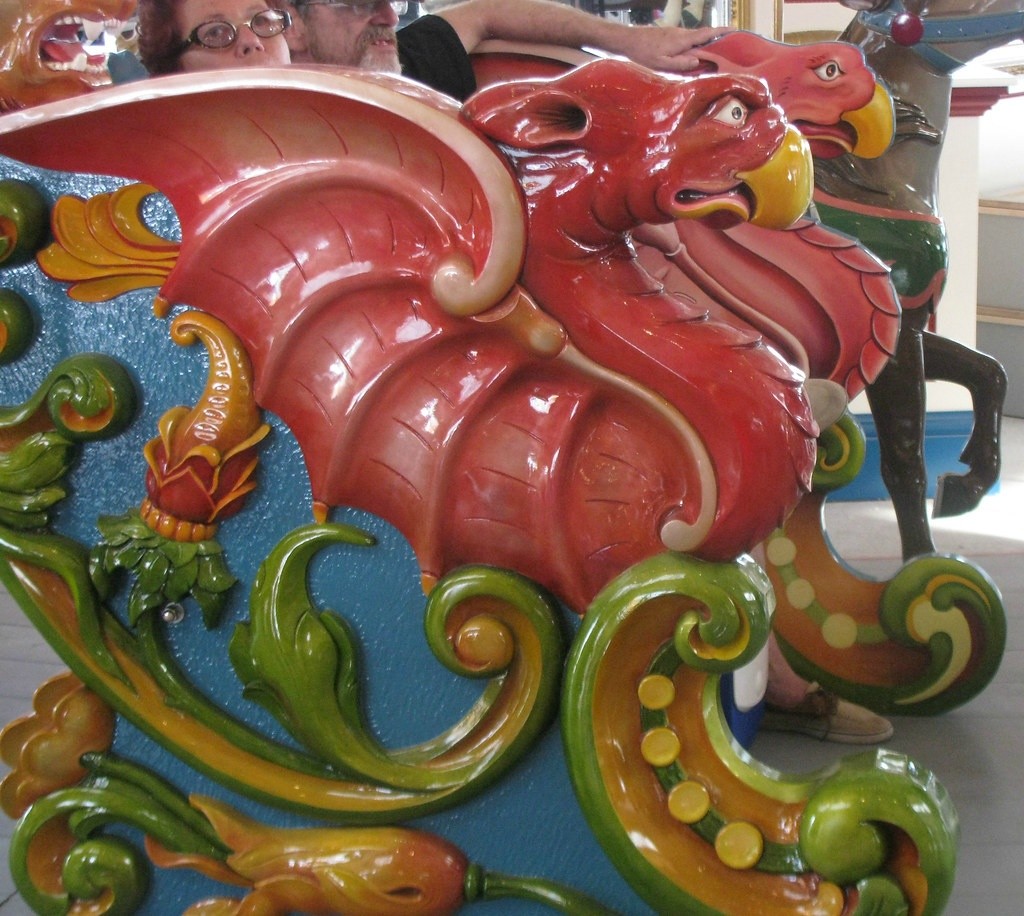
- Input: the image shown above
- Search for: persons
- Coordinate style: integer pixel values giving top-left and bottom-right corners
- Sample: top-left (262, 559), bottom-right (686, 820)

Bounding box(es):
top-left (136, 0), bottom-right (292, 77)
top-left (262, 0), bottom-right (896, 745)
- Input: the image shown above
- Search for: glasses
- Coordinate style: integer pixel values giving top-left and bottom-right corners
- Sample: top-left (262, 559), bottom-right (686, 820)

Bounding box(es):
top-left (298, 0), bottom-right (409, 16)
top-left (175, 9), bottom-right (292, 57)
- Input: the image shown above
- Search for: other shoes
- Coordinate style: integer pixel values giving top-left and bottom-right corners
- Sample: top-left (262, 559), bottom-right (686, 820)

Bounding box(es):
top-left (762, 681), bottom-right (893, 744)
